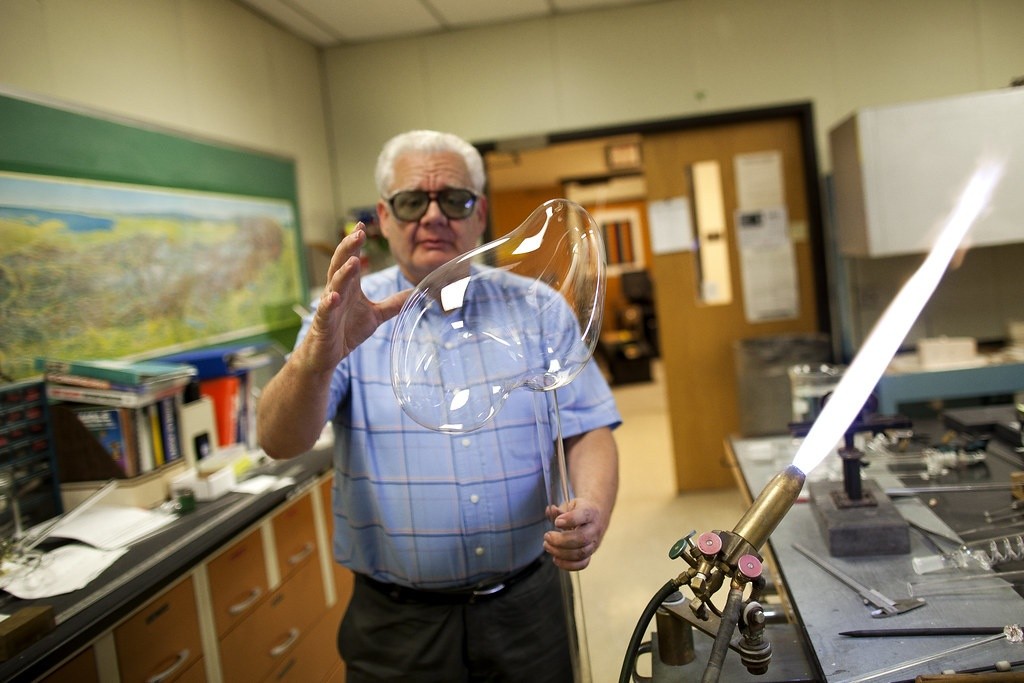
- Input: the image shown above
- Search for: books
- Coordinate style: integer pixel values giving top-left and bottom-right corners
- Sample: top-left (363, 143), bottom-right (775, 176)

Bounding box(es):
top-left (32, 340), bottom-right (274, 477)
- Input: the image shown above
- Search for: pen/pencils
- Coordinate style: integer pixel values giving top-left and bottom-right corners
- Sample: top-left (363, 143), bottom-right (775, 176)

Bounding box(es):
top-left (838, 628), bottom-right (1023, 637)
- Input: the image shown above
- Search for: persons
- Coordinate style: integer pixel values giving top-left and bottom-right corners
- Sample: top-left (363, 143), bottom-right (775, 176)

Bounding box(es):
top-left (255, 128), bottom-right (624, 683)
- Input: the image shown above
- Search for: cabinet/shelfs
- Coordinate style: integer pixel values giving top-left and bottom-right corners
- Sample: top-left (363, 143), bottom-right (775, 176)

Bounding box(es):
top-left (1, 439), bottom-right (355, 683)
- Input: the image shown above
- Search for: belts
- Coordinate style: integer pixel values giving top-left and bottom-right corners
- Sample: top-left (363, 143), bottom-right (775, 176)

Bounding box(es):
top-left (354, 550), bottom-right (549, 605)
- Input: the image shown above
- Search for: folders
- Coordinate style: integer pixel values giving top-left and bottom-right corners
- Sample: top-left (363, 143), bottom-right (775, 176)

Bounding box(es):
top-left (33, 356), bottom-right (197, 386)
top-left (164, 344), bottom-right (273, 378)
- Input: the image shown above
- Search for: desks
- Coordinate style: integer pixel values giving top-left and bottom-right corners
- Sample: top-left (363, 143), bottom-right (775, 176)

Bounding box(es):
top-left (714, 427), bottom-right (1024, 682)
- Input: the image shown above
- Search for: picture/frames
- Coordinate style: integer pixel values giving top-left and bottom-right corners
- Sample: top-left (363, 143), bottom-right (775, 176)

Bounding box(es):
top-left (0, 87), bottom-right (317, 398)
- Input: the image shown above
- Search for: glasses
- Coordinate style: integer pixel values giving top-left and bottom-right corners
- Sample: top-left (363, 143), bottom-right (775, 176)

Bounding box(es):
top-left (381, 187), bottom-right (483, 222)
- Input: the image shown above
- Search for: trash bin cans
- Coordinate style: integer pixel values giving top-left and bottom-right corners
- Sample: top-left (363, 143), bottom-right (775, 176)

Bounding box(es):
top-left (732, 334), bottom-right (832, 437)
